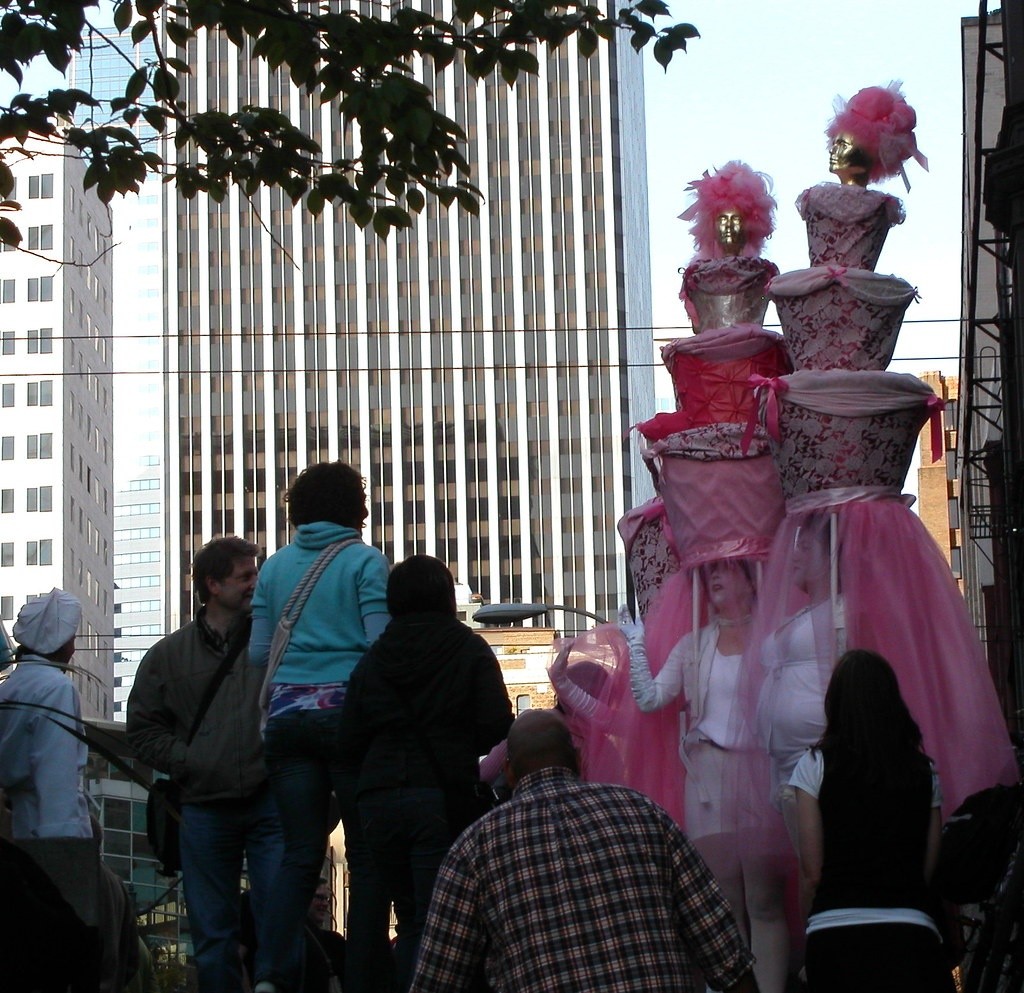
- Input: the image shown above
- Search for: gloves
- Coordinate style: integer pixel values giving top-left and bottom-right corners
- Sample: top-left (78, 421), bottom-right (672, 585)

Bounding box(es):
top-left (618, 605), bottom-right (655, 704)
top-left (548, 640), bottom-right (600, 721)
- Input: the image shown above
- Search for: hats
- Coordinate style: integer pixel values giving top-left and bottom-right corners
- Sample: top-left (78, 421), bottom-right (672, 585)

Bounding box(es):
top-left (12, 587), bottom-right (82, 654)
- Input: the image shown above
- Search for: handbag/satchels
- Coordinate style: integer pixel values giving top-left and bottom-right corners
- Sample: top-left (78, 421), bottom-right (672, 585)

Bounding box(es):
top-left (147, 778), bottom-right (181, 871)
top-left (258, 621), bottom-right (290, 714)
top-left (444, 782), bottom-right (498, 826)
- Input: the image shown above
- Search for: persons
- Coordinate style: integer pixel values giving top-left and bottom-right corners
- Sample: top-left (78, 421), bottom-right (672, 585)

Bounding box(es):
top-left (676, 159), bottom-right (778, 260)
top-left (239, 878), bottom-right (346, 992)
top-left (90, 813), bottom-right (139, 993)
top-left (790, 650), bottom-right (967, 993)
top-left (0, 587), bottom-right (94, 839)
top-left (824, 87), bottom-right (917, 187)
top-left (126, 539), bottom-right (304, 993)
top-left (246, 462), bottom-right (416, 974)
top-left (477, 662), bottom-right (622, 784)
top-left (410, 712), bottom-right (762, 993)
top-left (548, 509), bottom-right (861, 992)
top-left (127, 936), bottom-right (160, 993)
top-left (339, 554), bottom-right (515, 993)
